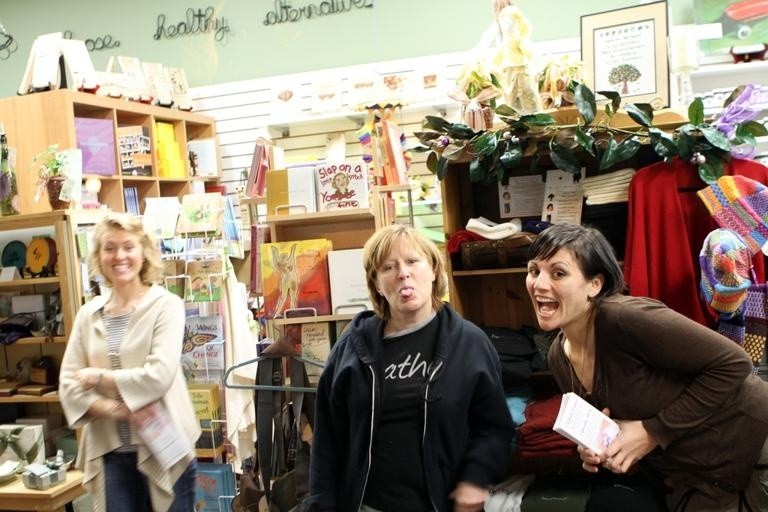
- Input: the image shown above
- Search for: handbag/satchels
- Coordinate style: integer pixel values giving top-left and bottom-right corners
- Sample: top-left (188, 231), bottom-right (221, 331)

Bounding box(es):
top-left (230, 338), bottom-right (315, 511)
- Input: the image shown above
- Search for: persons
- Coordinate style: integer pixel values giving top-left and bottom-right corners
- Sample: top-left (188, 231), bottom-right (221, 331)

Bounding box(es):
top-left (57, 213), bottom-right (202, 512)
top-left (526, 225), bottom-right (768, 511)
top-left (299, 222), bottom-right (514, 512)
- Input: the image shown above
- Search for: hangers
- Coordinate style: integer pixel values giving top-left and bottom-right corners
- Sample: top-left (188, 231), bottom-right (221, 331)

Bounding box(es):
top-left (224, 316), bottom-right (328, 394)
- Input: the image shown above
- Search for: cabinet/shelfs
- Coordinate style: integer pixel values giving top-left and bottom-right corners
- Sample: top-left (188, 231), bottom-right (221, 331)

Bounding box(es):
top-left (259, 184), bottom-right (414, 512)
top-left (442, 110), bottom-right (688, 512)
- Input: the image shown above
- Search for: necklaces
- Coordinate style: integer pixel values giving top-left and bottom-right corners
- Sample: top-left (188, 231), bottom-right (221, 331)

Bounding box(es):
top-left (569, 319), bottom-right (588, 396)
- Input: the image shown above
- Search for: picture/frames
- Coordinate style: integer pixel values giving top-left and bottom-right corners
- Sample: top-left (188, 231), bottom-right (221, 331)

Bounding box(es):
top-left (579, 1), bottom-right (670, 110)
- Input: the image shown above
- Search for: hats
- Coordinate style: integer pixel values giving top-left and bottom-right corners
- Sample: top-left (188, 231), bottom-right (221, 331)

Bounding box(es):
top-left (698, 227), bottom-right (753, 313)
top-left (696, 174), bottom-right (768, 258)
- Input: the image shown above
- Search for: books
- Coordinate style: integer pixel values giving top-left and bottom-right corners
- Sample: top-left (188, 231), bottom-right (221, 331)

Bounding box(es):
top-left (243, 120), bottom-right (408, 384)
top-left (73, 117), bottom-right (245, 511)
